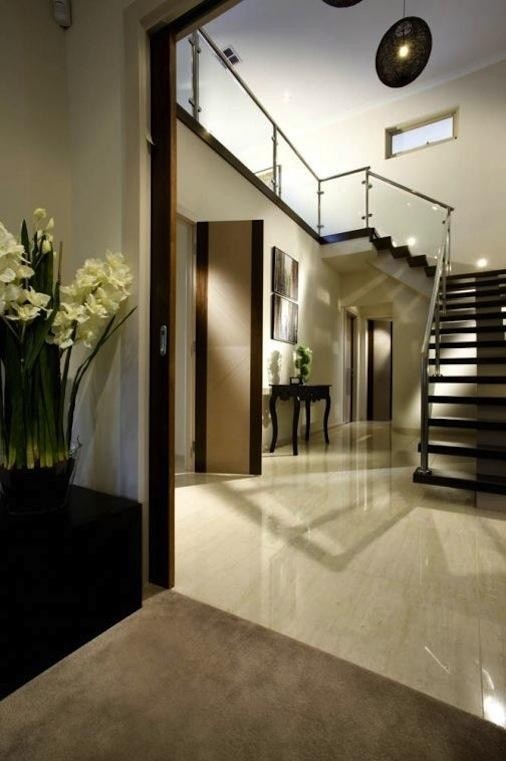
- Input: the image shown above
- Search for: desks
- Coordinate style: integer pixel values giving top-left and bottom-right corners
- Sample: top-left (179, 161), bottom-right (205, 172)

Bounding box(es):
top-left (267, 384), bottom-right (332, 455)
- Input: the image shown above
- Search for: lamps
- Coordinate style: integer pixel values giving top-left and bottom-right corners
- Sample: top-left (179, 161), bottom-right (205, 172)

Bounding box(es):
top-left (373, 0), bottom-right (434, 89)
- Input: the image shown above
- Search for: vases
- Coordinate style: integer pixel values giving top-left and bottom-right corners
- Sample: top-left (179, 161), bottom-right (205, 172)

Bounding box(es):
top-left (0, 456), bottom-right (76, 516)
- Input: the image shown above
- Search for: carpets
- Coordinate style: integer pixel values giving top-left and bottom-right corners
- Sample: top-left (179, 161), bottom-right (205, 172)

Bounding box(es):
top-left (0, 580), bottom-right (505, 761)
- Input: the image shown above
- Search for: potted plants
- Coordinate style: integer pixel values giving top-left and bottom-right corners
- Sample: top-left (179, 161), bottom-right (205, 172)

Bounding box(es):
top-left (293, 345), bottom-right (312, 384)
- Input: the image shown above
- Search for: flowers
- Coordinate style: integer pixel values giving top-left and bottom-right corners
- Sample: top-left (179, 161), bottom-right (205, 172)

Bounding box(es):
top-left (0, 205), bottom-right (139, 468)
